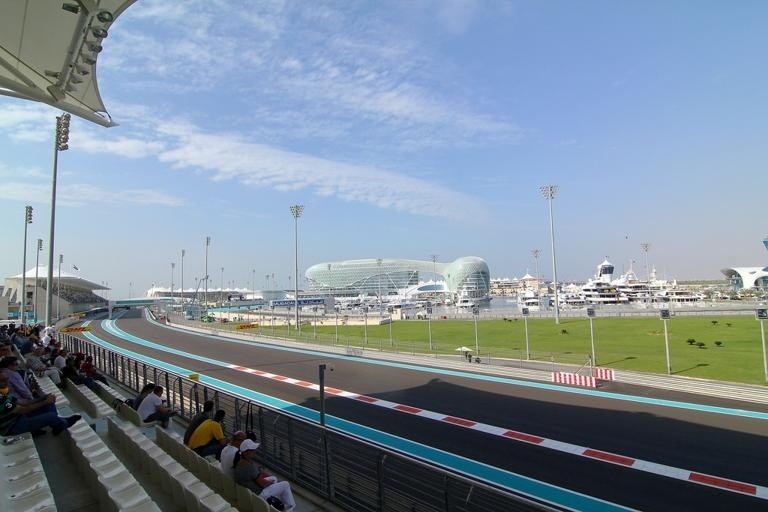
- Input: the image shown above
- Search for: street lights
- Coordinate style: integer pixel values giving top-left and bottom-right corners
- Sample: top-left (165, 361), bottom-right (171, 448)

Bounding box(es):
top-left (227, 306), bottom-right (317, 338)
top-left (386, 306), bottom-right (393, 347)
top-left (171, 237), bottom-right (209, 303)
top-left (427, 307), bottom-right (432, 351)
top-left (522, 308), bottom-right (529, 360)
top-left (290, 205), bottom-right (303, 330)
top-left (755, 308), bottom-right (767, 383)
top-left (431, 255), bottom-right (438, 308)
top-left (333, 307), bottom-right (339, 343)
top-left (362, 307), bottom-right (369, 343)
top-left (588, 309), bottom-right (595, 366)
top-left (46, 114), bottom-right (70, 328)
top-left (328, 264), bottom-right (332, 293)
top-left (531, 184), bottom-right (561, 322)
top-left (659, 310), bottom-right (672, 375)
top-left (20, 206), bottom-right (63, 324)
top-left (641, 242), bottom-right (652, 301)
top-left (473, 306), bottom-right (480, 354)
top-left (377, 257), bottom-right (382, 304)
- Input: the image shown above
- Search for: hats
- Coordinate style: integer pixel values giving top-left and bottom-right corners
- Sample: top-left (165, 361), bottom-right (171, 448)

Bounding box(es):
top-left (240, 438), bottom-right (261, 452)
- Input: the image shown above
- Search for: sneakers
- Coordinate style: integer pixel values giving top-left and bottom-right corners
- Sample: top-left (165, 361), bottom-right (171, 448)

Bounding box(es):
top-left (63, 414), bottom-right (81, 427)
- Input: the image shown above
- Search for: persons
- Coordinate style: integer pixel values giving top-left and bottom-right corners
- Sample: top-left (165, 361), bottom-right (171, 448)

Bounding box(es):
top-left (137, 386), bottom-right (173, 428)
top-left (0, 321), bottom-right (110, 438)
top-left (467, 353), bottom-right (472, 363)
top-left (183, 401), bottom-right (296, 512)
top-left (132, 383), bottom-right (155, 411)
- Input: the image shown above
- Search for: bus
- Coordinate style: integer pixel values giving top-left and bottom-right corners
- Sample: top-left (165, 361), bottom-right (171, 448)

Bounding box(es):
top-left (335, 303), bottom-right (426, 319)
top-left (269, 299), bottom-right (325, 313)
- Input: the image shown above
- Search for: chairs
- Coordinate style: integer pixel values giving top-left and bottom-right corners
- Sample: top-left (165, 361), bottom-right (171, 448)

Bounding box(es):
top-left (1, 342), bottom-right (130, 419)
top-left (0, 402), bottom-right (282, 511)
